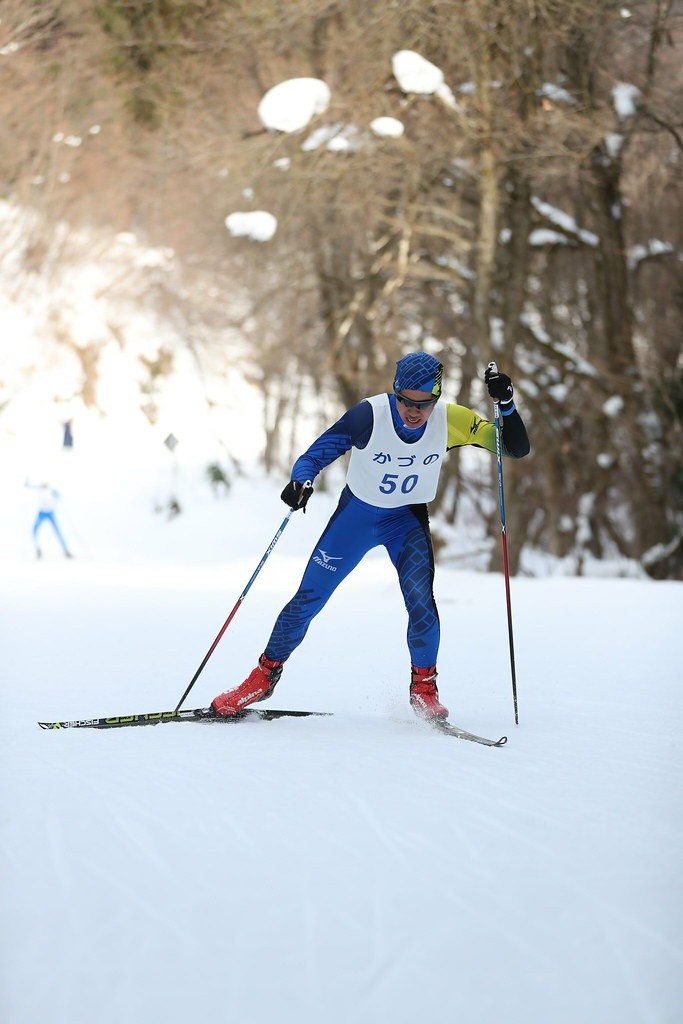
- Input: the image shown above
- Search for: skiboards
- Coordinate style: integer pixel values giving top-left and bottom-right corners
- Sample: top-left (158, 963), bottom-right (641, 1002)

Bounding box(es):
top-left (35, 705), bottom-right (510, 748)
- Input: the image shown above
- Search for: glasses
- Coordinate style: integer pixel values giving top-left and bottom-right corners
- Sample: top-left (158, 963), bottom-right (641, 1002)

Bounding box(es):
top-left (393, 383), bottom-right (438, 410)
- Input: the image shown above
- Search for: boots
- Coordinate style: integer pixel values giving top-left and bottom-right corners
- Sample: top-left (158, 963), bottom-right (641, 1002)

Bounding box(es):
top-left (410, 666), bottom-right (448, 720)
top-left (211, 651), bottom-right (285, 716)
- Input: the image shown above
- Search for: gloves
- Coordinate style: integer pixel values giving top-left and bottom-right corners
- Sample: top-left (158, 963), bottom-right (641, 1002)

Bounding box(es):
top-left (485, 368), bottom-right (513, 407)
top-left (281, 480), bottom-right (313, 510)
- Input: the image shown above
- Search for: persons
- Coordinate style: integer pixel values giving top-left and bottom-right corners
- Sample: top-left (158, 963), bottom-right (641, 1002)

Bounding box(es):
top-left (22, 475), bottom-right (72, 559)
top-left (211, 351), bottom-right (530, 721)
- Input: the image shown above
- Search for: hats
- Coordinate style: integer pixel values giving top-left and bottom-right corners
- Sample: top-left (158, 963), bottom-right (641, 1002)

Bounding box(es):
top-left (395, 352), bottom-right (443, 396)
